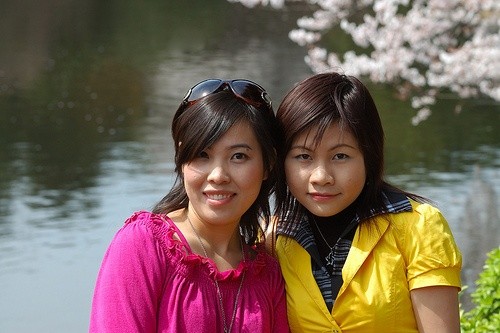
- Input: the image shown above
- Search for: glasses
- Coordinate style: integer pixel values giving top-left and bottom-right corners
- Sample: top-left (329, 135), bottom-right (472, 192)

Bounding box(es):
top-left (173, 79), bottom-right (273, 121)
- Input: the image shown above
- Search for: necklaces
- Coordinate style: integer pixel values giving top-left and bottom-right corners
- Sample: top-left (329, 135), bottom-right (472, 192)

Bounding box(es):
top-left (311, 212), bottom-right (356, 266)
top-left (183, 209), bottom-right (246, 333)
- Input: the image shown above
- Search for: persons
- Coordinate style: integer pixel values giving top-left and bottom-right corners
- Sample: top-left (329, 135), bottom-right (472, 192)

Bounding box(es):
top-left (89, 78), bottom-right (290, 333)
top-left (254, 72), bottom-right (463, 333)
